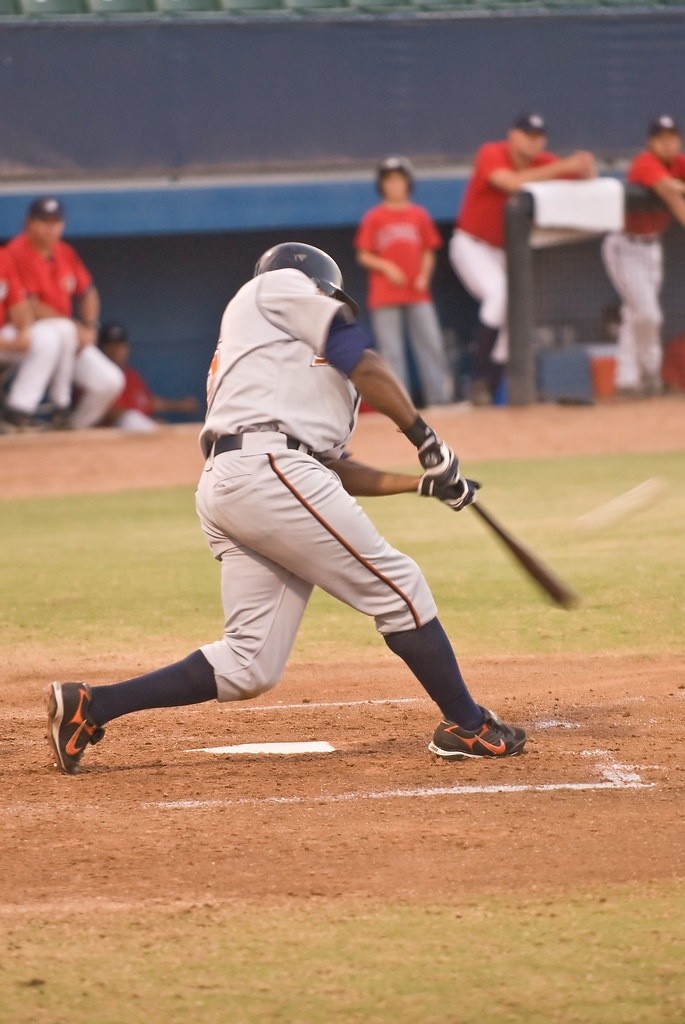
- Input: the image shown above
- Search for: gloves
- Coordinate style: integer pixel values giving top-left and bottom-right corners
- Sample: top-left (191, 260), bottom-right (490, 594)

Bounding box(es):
top-left (400, 416), bottom-right (460, 485)
top-left (418, 476), bottom-right (481, 513)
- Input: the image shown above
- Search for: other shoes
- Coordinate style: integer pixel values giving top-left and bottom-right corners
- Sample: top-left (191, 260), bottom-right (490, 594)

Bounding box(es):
top-left (472, 361), bottom-right (502, 403)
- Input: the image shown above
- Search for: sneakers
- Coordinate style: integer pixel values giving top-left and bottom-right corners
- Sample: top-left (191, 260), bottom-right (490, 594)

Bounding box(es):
top-left (46, 682), bottom-right (104, 776)
top-left (428, 705), bottom-right (527, 760)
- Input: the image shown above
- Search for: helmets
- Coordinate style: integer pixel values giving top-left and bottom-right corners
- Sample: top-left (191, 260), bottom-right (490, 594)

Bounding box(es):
top-left (254, 241), bottom-right (361, 320)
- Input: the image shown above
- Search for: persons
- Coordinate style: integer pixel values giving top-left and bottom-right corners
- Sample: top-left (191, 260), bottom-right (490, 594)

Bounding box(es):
top-left (47, 239), bottom-right (527, 778)
top-left (448, 108), bottom-right (598, 405)
top-left (352, 153), bottom-right (455, 410)
top-left (597, 115), bottom-right (685, 404)
top-left (1, 198), bottom-right (198, 436)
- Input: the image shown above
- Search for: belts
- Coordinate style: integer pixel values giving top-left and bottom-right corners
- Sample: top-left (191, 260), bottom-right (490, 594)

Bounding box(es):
top-left (207, 432), bottom-right (314, 456)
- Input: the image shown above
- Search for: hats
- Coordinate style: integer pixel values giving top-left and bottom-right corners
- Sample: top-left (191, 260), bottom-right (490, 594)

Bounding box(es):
top-left (99, 322), bottom-right (126, 342)
top-left (377, 157), bottom-right (415, 193)
top-left (30, 198), bottom-right (61, 219)
top-left (648, 115), bottom-right (678, 136)
top-left (511, 114), bottom-right (547, 134)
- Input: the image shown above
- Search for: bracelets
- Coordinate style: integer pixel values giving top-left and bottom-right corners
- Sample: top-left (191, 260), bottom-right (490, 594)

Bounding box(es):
top-left (400, 418), bottom-right (429, 448)
top-left (84, 320), bottom-right (102, 331)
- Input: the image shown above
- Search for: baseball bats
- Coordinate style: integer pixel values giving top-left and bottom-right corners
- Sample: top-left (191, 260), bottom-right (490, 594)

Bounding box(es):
top-left (426, 451), bottom-right (576, 612)
